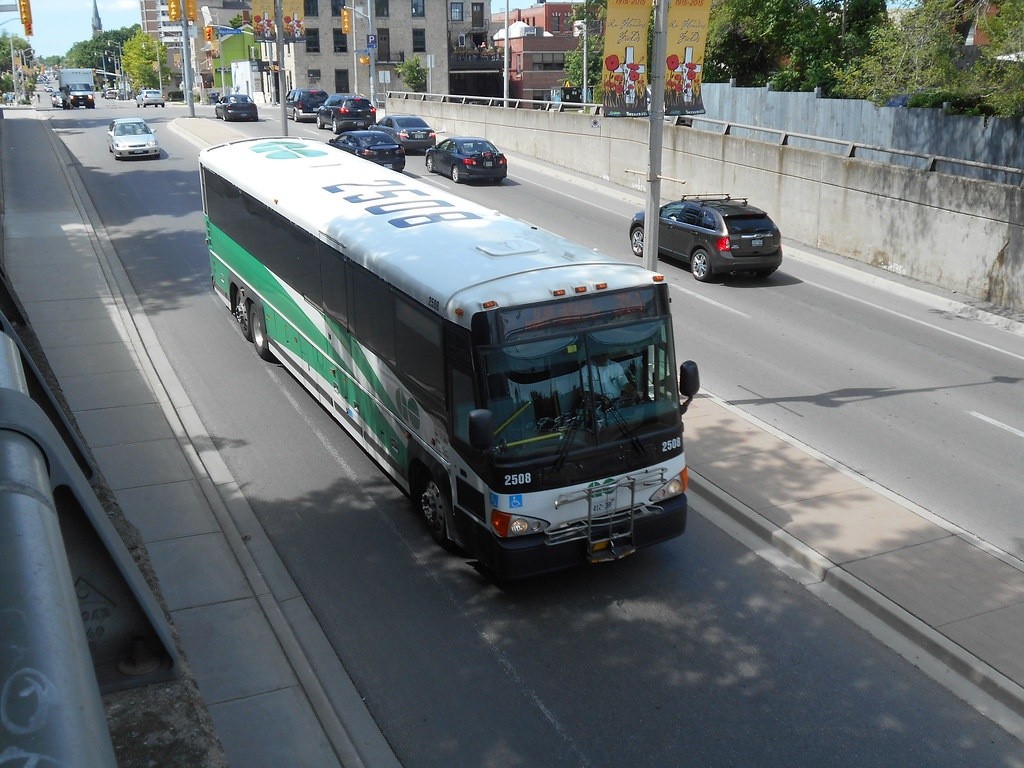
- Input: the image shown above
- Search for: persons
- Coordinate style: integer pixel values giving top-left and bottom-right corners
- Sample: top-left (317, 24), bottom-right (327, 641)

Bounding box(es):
top-left (577, 358), bottom-right (634, 408)
top-left (2, 92), bottom-right (15, 106)
top-left (37, 93), bottom-right (40, 103)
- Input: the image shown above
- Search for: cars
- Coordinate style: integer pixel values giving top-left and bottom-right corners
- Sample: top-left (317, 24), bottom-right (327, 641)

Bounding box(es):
top-left (425, 137), bottom-right (508, 184)
top-left (102, 87), bottom-right (116, 99)
top-left (106, 117), bottom-right (161, 161)
top-left (135, 89), bottom-right (165, 108)
top-left (214, 94), bottom-right (258, 122)
top-left (326, 129), bottom-right (406, 173)
top-left (368, 115), bottom-right (437, 153)
top-left (285, 88), bottom-right (329, 122)
top-left (315, 93), bottom-right (378, 134)
top-left (42, 74), bottom-right (63, 107)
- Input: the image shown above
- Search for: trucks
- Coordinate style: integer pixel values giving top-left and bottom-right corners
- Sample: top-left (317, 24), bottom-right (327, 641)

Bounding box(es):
top-left (56, 68), bottom-right (95, 111)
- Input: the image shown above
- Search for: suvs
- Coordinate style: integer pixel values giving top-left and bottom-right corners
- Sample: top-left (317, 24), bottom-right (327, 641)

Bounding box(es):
top-left (628, 193), bottom-right (783, 282)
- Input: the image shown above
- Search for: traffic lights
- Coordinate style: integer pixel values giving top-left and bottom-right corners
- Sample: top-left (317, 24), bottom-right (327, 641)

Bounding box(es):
top-left (203, 27), bottom-right (213, 42)
top-left (25, 21), bottom-right (32, 36)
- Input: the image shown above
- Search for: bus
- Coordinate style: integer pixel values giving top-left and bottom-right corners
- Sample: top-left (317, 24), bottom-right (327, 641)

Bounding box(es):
top-left (198, 135), bottom-right (702, 584)
